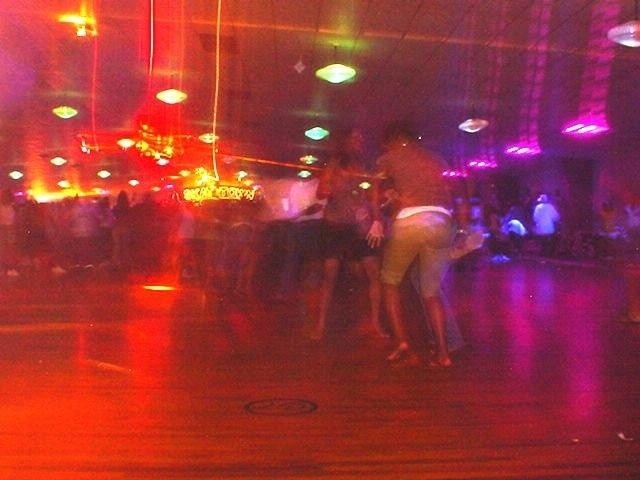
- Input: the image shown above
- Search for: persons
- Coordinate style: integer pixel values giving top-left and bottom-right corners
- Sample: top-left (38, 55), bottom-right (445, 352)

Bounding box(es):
top-left (1, 185), bottom-right (639, 283)
top-left (365, 122), bottom-right (471, 371)
top-left (305, 123), bottom-right (393, 344)
